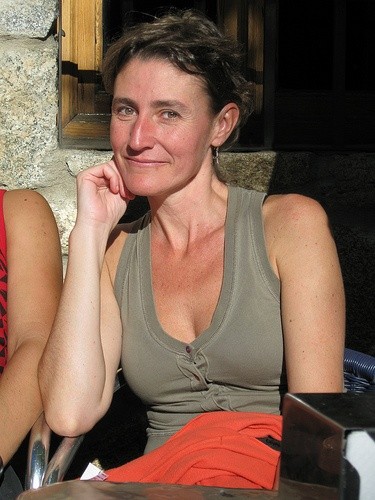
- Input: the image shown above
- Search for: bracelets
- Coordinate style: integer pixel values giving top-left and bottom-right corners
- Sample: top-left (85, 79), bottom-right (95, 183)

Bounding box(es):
top-left (0, 456), bottom-right (4, 475)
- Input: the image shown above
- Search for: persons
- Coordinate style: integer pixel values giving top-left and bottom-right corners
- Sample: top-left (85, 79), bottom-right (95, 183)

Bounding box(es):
top-left (38, 11), bottom-right (346, 491)
top-left (0, 189), bottom-right (63, 500)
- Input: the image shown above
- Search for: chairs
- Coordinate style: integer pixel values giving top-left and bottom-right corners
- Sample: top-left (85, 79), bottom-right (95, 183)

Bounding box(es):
top-left (25, 348), bottom-right (375, 489)
